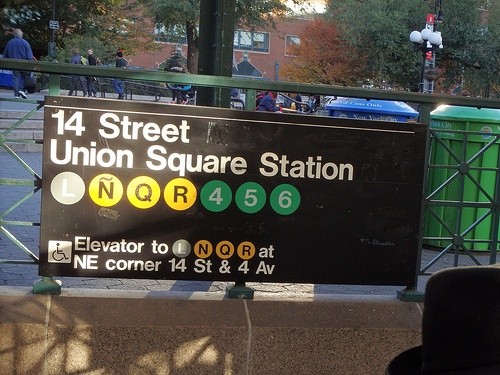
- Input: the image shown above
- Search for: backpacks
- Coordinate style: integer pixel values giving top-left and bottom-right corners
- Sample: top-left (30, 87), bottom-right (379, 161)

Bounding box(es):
top-left (81, 55), bottom-right (89, 65)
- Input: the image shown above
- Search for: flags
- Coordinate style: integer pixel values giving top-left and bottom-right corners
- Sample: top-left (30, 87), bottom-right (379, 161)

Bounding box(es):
top-left (426, 14), bottom-right (434, 60)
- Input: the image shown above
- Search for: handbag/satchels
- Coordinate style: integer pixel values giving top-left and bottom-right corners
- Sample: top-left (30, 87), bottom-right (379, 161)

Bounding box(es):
top-left (24, 76), bottom-right (37, 94)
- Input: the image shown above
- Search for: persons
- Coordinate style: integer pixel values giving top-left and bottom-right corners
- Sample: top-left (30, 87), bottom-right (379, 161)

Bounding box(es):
top-left (168, 55), bottom-right (320, 114)
top-left (113, 49), bottom-right (128, 100)
top-left (68, 48), bottom-right (87, 97)
top-left (4, 29), bottom-right (33, 99)
top-left (461, 90), bottom-right (497, 99)
top-left (86, 47), bottom-right (97, 97)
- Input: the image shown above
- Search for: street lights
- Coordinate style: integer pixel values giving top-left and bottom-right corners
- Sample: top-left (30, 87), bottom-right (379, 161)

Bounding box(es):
top-left (410, 28), bottom-right (442, 92)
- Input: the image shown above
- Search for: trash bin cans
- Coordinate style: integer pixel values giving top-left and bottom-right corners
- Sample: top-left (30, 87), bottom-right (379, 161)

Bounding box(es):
top-left (325, 96), bottom-right (500, 255)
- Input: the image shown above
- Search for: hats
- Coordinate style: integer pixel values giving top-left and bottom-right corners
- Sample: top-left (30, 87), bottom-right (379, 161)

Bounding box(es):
top-left (384, 263), bottom-right (500, 375)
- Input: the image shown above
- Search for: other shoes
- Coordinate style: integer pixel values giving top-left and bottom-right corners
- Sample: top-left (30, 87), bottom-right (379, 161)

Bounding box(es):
top-left (18, 90), bottom-right (28, 99)
top-left (14, 93), bottom-right (21, 98)
